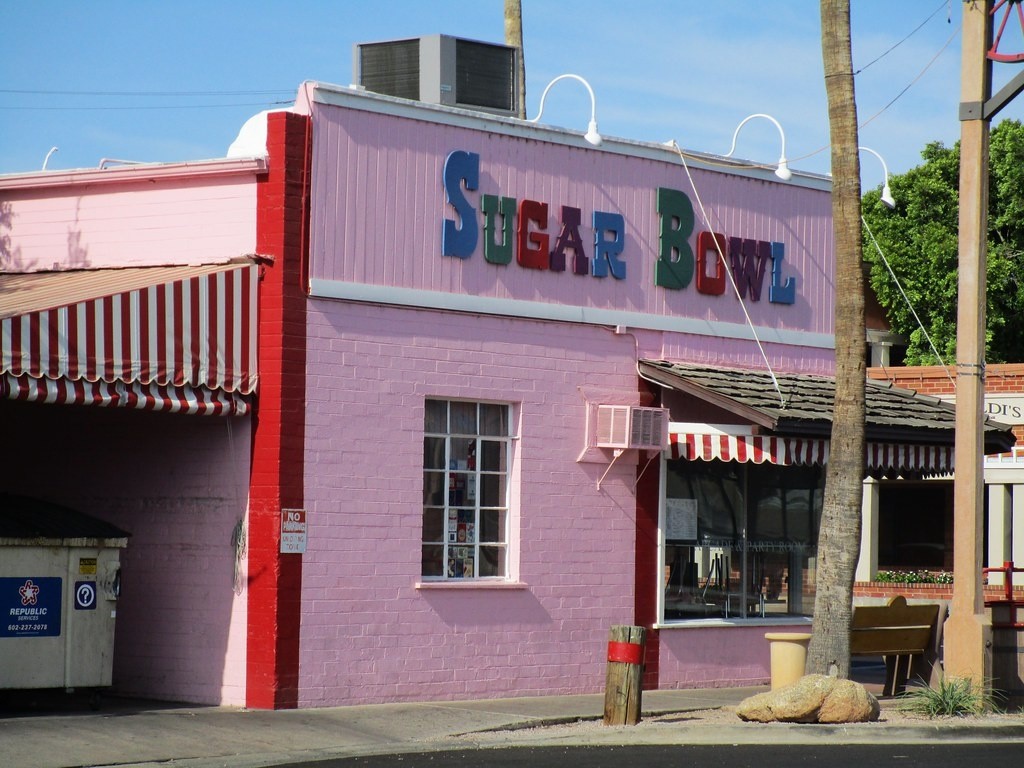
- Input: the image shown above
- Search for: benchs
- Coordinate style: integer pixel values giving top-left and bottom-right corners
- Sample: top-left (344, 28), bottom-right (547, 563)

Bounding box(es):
top-left (846, 593), bottom-right (942, 697)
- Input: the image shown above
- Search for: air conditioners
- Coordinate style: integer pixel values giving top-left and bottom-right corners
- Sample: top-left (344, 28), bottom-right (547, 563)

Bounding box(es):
top-left (594, 404), bottom-right (671, 455)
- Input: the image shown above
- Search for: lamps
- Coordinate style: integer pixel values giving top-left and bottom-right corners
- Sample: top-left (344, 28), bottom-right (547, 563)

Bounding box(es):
top-left (721, 113), bottom-right (794, 182)
top-left (531, 71), bottom-right (605, 148)
top-left (857, 145), bottom-right (897, 208)
